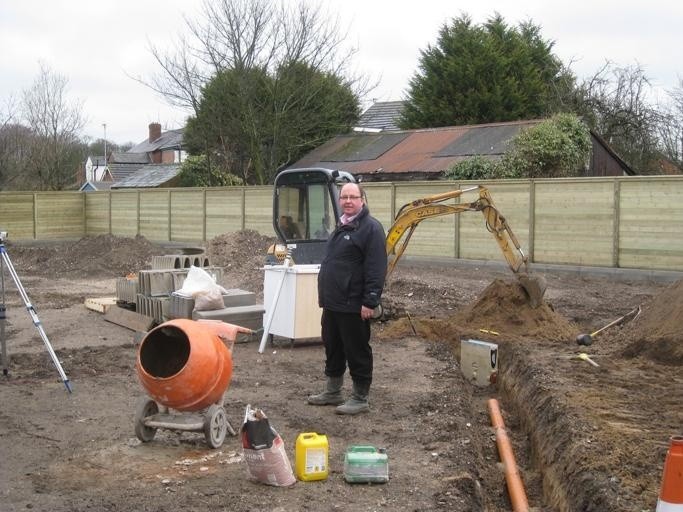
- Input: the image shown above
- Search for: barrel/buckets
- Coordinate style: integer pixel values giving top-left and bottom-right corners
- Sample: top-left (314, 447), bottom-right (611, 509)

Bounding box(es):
top-left (342, 444), bottom-right (389, 484)
top-left (295, 431), bottom-right (329, 480)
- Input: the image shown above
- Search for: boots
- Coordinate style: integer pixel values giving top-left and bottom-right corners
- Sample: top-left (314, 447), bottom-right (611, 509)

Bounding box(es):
top-left (305, 373), bottom-right (346, 407)
top-left (334, 377), bottom-right (373, 416)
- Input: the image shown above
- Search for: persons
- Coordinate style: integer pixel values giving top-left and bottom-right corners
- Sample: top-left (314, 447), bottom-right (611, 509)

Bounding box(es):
top-left (307, 182), bottom-right (388, 415)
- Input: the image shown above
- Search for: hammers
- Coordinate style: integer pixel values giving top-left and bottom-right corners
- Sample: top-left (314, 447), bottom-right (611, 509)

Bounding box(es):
top-left (576, 308), bottom-right (636, 345)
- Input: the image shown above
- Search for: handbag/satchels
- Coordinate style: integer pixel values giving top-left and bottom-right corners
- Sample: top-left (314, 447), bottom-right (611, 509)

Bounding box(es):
top-left (238, 402), bottom-right (297, 489)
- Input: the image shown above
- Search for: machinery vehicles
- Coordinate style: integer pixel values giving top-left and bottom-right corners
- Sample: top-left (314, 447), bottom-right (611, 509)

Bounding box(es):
top-left (264, 168), bottom-right (548, 310)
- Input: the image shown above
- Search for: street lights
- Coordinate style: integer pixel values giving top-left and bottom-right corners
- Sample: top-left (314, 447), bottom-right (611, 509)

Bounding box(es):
top-left (103, 124), bottom-right (106, 170)
